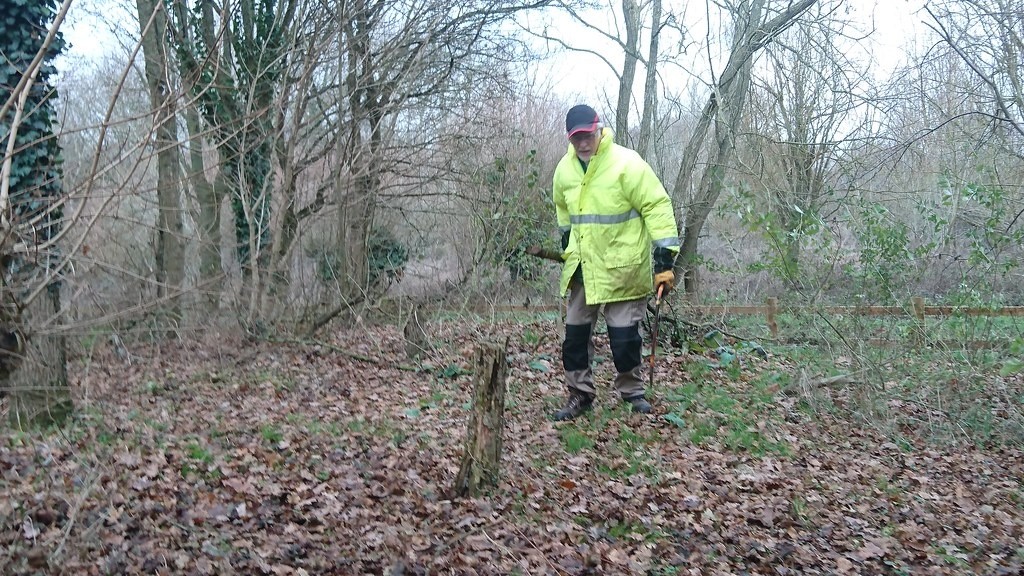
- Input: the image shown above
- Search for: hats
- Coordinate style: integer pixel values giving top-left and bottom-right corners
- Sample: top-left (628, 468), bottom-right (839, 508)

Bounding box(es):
top-left (565, 104), bottom-right (600, 139)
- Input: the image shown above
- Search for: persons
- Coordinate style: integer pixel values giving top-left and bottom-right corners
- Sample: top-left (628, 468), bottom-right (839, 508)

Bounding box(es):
top-left (552, 106), bottom-right (680, 420)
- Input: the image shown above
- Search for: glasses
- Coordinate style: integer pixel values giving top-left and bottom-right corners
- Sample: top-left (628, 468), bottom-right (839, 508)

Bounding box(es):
top-left (568, 127), bottom-right (602, 142)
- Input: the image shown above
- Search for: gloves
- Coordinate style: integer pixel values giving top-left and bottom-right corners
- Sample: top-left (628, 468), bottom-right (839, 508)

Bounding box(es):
top-left (653, 246), bottom-right (676, 298)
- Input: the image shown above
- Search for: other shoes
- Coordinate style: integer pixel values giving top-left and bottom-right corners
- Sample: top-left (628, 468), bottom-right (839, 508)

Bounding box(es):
top-left (551, 391), bottom-right (596, 423)
top-left (622, 398), bottom-right (653, 414)
